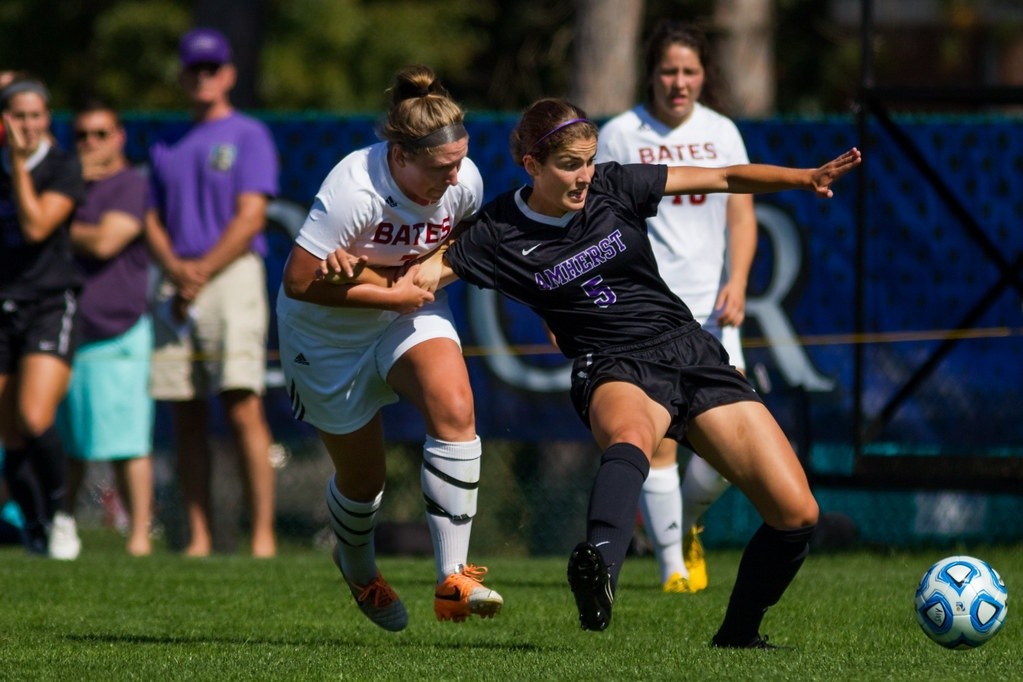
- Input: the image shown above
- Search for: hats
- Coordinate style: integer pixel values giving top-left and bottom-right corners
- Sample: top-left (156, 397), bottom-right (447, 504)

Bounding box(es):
top-left (180, 29), bottom-right (233, 66)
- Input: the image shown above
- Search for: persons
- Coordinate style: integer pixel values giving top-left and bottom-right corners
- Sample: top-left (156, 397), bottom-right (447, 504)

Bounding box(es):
top-left (586, 21), bottom-right (759, 593)
top-left (0, 70), bottom-right (86, 561)
top-left (71, 103), bottom-right (154, 557)
top-left (140, 31), bottom-right (279, 559)
top-left (312, 96), bottom-right (864, 650)
top-left (277, 65), bottom-right (504, 632)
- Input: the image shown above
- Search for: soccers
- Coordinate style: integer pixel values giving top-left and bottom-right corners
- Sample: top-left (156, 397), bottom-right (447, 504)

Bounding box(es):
top-left (914, 555), bottom-right (1009, 650)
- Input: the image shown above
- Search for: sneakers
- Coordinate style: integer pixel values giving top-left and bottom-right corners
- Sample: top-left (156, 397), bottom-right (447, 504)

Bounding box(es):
top-left (565, 540), bottom-right (615, 633)
top-left (330, 541), bottom-right (409, 633)
top-left (682, 521), bottom-right (709, 592)
top-left (47, 509), bottom-right (83, 562)
top-left (431, 571), bottom-right (503, 623)
top-left (660, 572), bottom-right (690, 594)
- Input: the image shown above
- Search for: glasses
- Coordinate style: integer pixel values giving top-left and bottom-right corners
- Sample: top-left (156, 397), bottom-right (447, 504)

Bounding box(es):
top-left (71, 126), bottom-right (112, 140)
